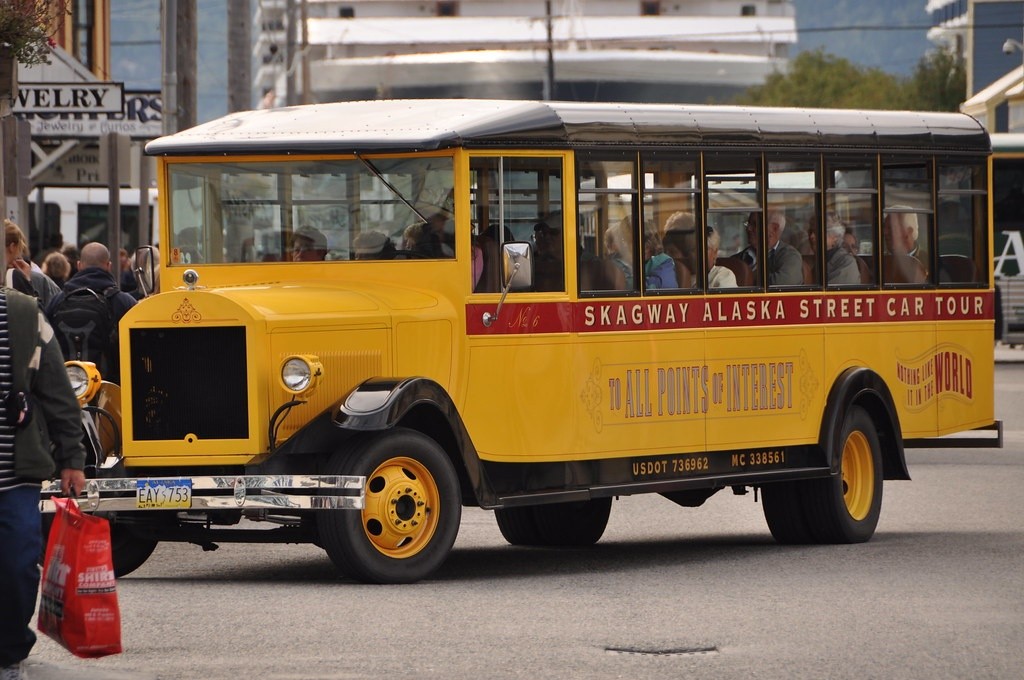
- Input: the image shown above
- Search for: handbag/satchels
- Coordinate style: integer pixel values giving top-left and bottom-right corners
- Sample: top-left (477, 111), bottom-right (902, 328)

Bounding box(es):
top-left (37, 494), bottom-right (122, 659)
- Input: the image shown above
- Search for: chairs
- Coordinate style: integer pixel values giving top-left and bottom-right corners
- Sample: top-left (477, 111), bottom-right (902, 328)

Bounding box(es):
top-left (473, 240), bottom-right (971, 297)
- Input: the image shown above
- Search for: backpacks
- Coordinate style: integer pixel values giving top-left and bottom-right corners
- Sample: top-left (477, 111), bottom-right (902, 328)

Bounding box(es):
top-left (50, 285), bottom-right (121, 383)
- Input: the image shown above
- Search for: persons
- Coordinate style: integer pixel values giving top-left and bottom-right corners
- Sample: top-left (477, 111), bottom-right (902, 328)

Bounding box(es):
top-left (0, 221), bottom-right (158, 386)
top-left (0, 290), bottom-right (86, 680)
top-left (290, 203), bottom-right (934, 292)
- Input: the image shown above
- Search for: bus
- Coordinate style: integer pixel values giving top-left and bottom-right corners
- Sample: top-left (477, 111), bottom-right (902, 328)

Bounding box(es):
top-left (37, 99), bottom-right (1003, 586)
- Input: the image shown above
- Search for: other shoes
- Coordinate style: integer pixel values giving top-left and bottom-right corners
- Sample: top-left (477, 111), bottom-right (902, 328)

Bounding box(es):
top-left (0, 662), bottom-right (26, 680)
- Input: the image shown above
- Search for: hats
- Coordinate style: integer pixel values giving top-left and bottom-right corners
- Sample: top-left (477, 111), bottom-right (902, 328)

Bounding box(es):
top-left (533, 212), bottom-right (562, 229)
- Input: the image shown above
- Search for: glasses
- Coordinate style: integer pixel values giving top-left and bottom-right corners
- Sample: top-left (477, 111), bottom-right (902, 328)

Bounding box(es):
top-left (537, 230), bottom-right (559, 237)
top-left (742, 221), bottom-right (755, 229)
